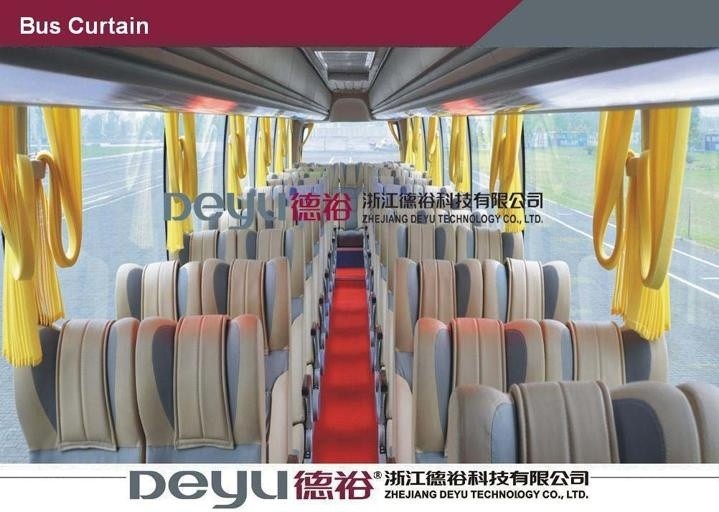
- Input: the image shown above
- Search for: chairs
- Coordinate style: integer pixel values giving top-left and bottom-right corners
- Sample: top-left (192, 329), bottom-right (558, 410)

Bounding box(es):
top-left (677, 379), bottom-right (719, 462)
top-left (134, 313), bottom-right (269, 463)
top-left (12, 317), bottom-right (141, 465)
top-left (115, 259), bottom-right (202, 321)
top-left (439, 376), bottom-right (700, 464)
top-left (542, 314), bottom-right (671, 385)
top-left (186, 160), bottom-right (572, 462)
